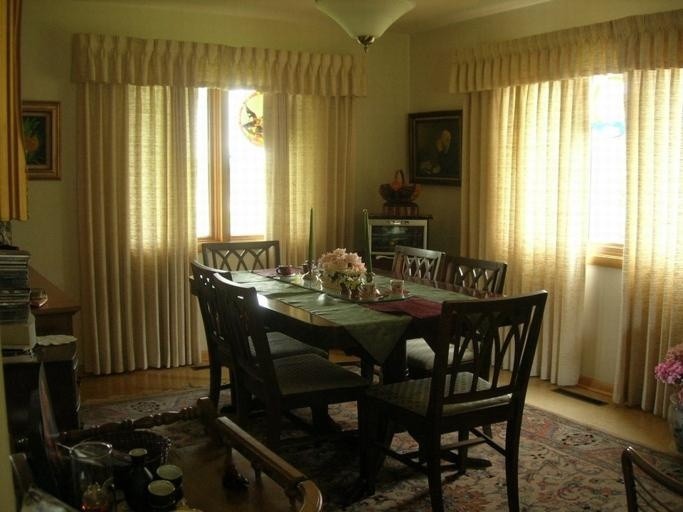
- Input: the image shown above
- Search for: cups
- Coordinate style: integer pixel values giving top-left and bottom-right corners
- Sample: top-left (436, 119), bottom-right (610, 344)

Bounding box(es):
top-left (275, 263), bottom-right (292, 276)
top-left (358, 282), bottom-right (374, 300)
top-left (145, 479), bottom-right (177, 511)
top-left (390, 280), bottom-right (404, 297)
top-left (70, 441), bottom-right (113, 512)
top-left (156, 463), bottom-right (184, 502)
top-left (302, 258), bottom-right (323, 277)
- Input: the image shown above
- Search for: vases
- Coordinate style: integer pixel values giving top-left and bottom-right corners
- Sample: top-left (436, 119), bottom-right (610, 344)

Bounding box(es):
top-left (381, 202), bottom-right (418, 218)
top-left (317, 272), bottom-right (357, 292)
top-left (671, 426), bottom-right (683, 454)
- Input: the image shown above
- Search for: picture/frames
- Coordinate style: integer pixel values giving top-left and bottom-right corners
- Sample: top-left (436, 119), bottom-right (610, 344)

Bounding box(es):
top-left (406, 106), bottom-right (461, 188)
top-left (18, 96), bottom-right (63, 183)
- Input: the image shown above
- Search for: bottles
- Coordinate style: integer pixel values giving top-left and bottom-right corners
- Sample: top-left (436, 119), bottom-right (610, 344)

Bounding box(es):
top-left (302, 262), bottom-right (317, 287)
top-left (123, 447), bottom-right (153, 511)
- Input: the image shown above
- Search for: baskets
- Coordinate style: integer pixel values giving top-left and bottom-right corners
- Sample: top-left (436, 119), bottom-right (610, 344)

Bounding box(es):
top-left (80, 429), bottom-right (172, 481)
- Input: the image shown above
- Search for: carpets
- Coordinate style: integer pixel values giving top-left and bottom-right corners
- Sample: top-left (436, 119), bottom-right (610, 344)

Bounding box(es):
top-left (81, 381), bottom-right (683, 511)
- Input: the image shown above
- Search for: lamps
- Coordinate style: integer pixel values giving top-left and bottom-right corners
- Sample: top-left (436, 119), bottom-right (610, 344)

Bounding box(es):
top-left (312, 0), bottom-right (419, 55)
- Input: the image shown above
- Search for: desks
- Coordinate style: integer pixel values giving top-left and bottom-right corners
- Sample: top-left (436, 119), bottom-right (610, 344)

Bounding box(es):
top-left (220, 266), bottom-right (491, 507)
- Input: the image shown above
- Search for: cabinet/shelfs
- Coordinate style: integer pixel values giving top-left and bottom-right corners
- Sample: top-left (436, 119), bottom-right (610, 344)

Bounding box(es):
top-left (25, 262), bottom-right (80, 335)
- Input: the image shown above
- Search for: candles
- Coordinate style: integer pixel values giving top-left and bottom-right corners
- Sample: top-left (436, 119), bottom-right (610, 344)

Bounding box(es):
top-left (307, 207), bottom-right (314, 262)
top-left (360, 206), bottom-right (375, 281)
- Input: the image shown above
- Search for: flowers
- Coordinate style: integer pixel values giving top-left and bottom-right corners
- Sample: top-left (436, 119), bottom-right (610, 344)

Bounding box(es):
top-left (650, 342), bottom-right (683, 416)
top-left (316, 244), bottom-right (366, 283)
top-left (378, 168), bottom-right (421, 202)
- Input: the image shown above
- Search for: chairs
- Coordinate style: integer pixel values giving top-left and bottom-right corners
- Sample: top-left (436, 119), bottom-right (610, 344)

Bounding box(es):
top-left (200, 240), bottom-right (333, 407)
top-left (391, 244), bottom-right (443, 287)
top-left (612, 439), bottom-right (683, 511)
top-left (209, 266), bottom-right (370, 481)
top-left (188, 258), bottom-right (332, 432)
top-left (362, 287), bottom-right (553, 511)
top-left (403, 256), bottom-right (507, 441)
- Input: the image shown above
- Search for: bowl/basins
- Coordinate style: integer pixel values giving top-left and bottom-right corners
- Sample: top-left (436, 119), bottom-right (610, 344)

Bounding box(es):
top-left (77, 428), bottom-right (172, 489)
